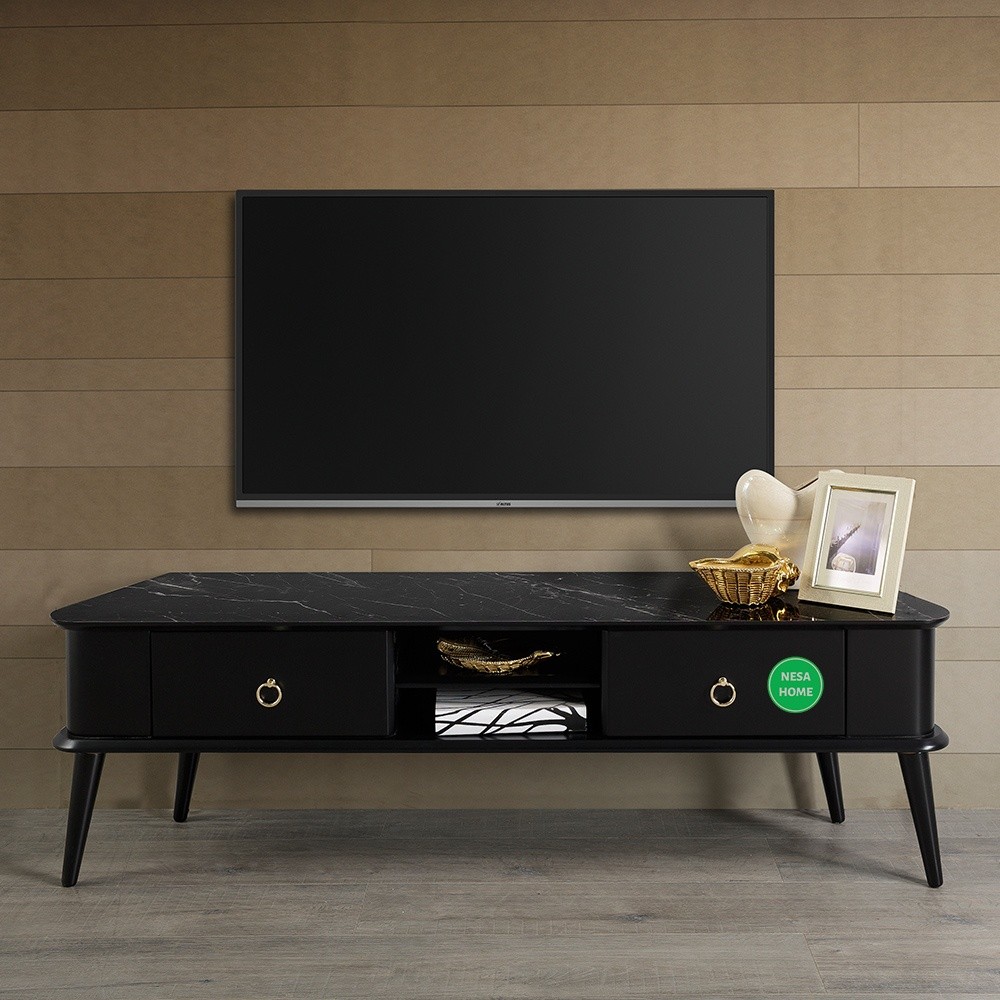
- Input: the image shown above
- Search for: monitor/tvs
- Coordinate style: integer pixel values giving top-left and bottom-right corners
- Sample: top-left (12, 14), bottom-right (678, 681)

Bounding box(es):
top-left (234, 188), bottom-right (775, 509)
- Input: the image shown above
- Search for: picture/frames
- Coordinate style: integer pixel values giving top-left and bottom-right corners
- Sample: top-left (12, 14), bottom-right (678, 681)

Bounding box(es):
top-left (800, 469), bottom-right (918, 613)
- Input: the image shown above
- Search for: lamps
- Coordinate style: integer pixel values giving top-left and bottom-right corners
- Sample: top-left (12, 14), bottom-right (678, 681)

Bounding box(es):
top-left (734, 469), bottom-right (817, 549)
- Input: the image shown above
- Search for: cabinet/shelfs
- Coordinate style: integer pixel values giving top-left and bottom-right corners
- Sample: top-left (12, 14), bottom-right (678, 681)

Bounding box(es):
top-left (51, 572), bottom-right (949, 889)
